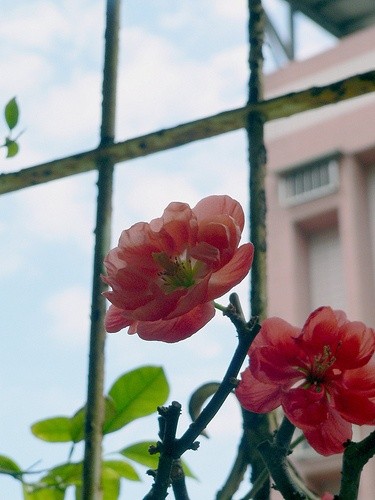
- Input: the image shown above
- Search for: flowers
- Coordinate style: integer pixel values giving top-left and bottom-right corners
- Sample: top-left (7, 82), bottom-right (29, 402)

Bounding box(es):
top-left (96, 191), bottom-right (375, 500)
